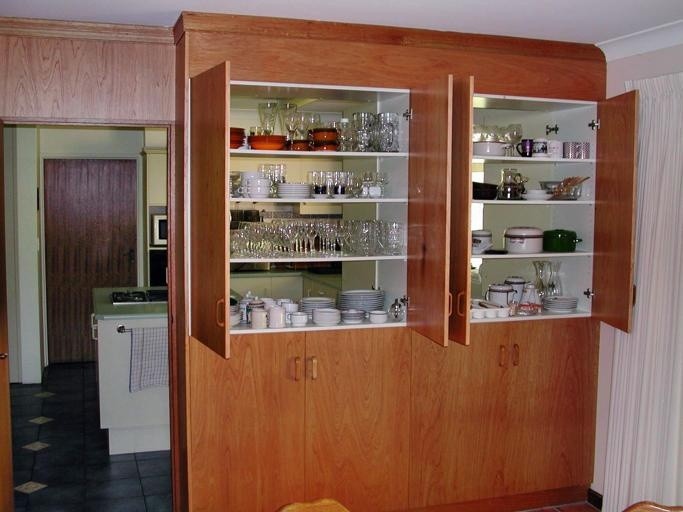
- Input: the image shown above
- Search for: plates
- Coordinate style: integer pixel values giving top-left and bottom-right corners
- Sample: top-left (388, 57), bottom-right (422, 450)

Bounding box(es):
top-left (230, 127), bottom-right (245, 148)
top-left (300, 289), bottom-right (384, 326)
top-left (471, 308), bottom-right (508, 319)
top-left (524, 190), bottom-right (555, 201)
top-left (278, 182), bottom-right (310, 199)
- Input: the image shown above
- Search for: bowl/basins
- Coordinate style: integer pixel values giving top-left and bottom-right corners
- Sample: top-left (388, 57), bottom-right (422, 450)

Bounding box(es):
top-left (313, 129), bottom-right (337, 150)
top-left (236, 173), bottom-right (273, 198)
top-left (249, 135), bottom-right (287, 150)
top-left (545, 297), bottom-right (577, 314)
top-left (538, 181), bottom-right (581, 199)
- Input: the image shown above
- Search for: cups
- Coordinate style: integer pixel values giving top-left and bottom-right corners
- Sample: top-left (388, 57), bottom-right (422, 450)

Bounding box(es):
top-left (240, 294), bottom-right (308, 328)
top-left (365, 310), bottom-right (388, 323)
top-left (473, 230), bottom-right (492, 253)
top-left (338, 112), bottom-right (398, 151)
top-left (341, 218), bottom-right (402, 255)
top-left (516, 139), bottom-right (589, 159)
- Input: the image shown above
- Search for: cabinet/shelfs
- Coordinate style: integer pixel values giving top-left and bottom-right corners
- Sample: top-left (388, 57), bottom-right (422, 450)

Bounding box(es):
top-left (171, 11), bottom-right (641, 510)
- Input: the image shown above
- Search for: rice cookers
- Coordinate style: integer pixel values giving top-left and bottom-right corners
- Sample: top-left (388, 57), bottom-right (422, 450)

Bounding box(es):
top-left (503, 226), bottom-right (544, 254)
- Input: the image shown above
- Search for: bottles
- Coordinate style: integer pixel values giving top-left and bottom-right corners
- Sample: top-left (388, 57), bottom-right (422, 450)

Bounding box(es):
top-left (307, 170), bottom-right (354, 195)
top-left (388, 299), bottom-right (404, 322)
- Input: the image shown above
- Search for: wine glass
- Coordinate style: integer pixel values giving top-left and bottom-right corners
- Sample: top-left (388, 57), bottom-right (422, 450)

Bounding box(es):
top-left (507, 123), bottom-right (523, 156)
top-left (362, 169), bottom-right (389, 200)
top-left (259, 103), bottom-right (321, 151)
top-left (231, 220), bottom-right (338, 256)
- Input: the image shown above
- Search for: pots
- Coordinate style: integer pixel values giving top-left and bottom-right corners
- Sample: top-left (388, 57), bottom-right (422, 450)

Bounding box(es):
top-left (473, 140), bottom-right (512, 155)
top-left (544, 230), bottom-right (582, 252)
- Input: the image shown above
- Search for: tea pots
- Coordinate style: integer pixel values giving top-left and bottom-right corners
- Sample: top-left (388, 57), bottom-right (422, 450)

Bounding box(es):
top-left (495, 168), bottom-right (524, 200)
top-left (487, 283), bottom-right (541, 316)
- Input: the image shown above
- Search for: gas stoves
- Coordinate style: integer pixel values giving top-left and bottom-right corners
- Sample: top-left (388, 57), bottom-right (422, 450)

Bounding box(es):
top-left (111, 290), bottom-right (168, 305)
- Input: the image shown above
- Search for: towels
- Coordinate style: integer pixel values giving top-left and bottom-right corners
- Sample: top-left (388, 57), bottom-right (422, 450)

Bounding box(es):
top-left (128, 327), bottom-right (170, 394)
